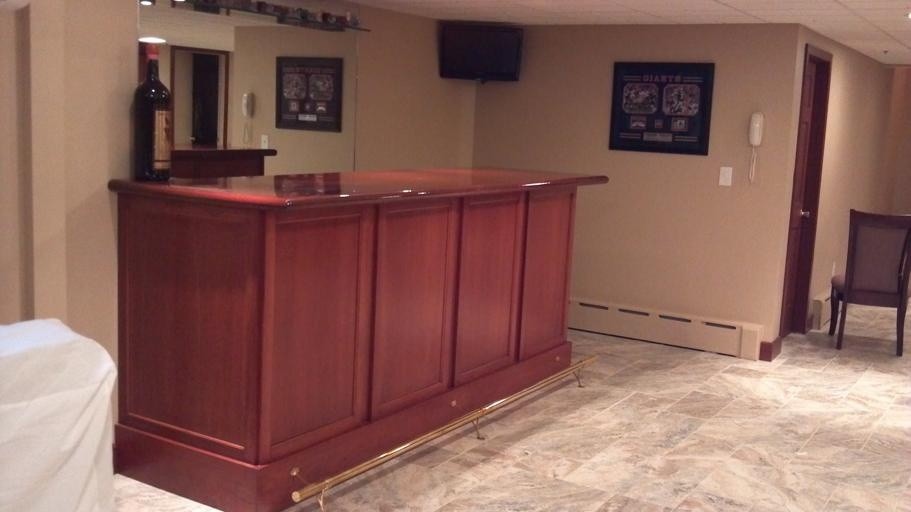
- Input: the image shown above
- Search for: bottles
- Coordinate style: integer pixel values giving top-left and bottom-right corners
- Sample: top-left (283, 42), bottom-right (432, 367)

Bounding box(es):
top-left (130, 41), bottom-right (175, 180)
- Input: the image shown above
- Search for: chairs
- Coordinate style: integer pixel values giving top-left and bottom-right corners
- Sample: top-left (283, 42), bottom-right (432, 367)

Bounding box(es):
top-left (829, 206), bottom-right (909, 362)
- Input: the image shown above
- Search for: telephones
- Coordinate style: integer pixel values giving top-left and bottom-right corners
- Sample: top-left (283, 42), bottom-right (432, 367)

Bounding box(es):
top-left (750, 111), bottom-right (764, 147)
top-left (242, 92), bottom-right (254, 119)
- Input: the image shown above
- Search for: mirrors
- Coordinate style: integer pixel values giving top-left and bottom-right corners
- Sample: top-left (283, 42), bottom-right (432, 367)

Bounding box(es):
top-left (137, 1), bottom-right (357, 190)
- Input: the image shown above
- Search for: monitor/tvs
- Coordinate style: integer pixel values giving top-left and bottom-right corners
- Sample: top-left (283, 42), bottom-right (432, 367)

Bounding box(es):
top-left (437, 22), bottom-right (525, 81)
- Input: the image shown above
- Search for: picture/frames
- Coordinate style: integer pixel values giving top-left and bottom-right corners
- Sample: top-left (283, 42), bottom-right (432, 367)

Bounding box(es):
top-left (608, 62), bottom-right (714, 157)
top-left (271, 55), bottom-right (347, 135)
